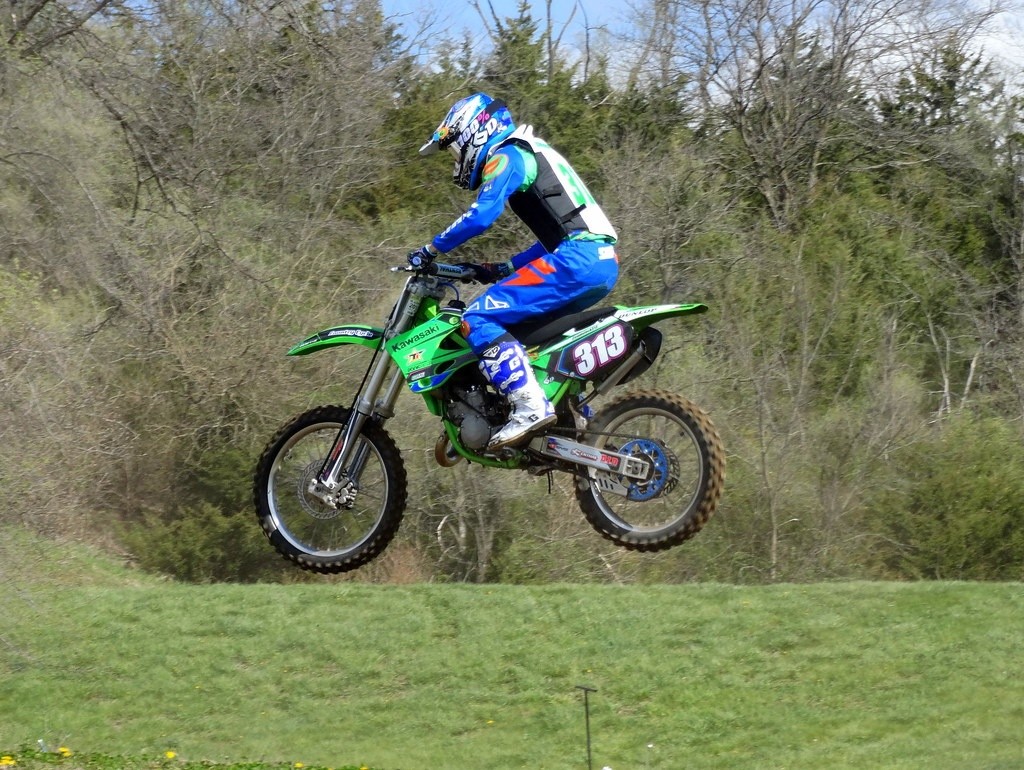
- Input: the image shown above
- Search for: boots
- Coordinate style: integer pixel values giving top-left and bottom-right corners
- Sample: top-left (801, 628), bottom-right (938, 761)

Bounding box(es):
top-left (479, 339), bottom-right (557, 452)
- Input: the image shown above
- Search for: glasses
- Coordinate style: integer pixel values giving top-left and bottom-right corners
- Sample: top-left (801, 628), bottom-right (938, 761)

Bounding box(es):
top-left (447, 141), bottom-right (462, 163)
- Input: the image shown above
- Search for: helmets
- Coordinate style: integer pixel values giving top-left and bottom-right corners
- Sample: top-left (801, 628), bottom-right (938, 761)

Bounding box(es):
top-left (419, 93), bottom-right (515, 190)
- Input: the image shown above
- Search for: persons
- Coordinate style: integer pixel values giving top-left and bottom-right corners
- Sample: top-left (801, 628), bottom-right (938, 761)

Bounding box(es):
top-left (416, 93), bottom-right (618, 451)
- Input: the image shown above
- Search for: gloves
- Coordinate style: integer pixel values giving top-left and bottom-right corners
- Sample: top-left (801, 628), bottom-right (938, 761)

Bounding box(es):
top-left (407, 245), bottom-right (437, 267)
top-left (481, 262), bottom-right (511, 280)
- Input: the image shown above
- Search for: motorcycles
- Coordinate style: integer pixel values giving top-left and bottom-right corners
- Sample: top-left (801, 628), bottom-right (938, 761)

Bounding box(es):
top-left (249, 255), bottom-right (725, 572)
